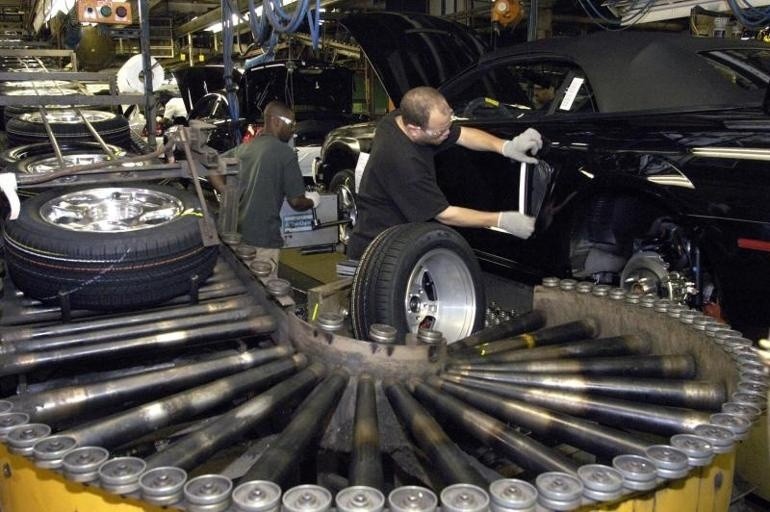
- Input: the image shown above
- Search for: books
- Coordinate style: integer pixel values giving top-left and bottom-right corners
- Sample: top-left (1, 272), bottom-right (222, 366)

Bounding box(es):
top-left (297, 243), bottom-right (335, 255)
top-left (334, 259), bottom-right (359, 278)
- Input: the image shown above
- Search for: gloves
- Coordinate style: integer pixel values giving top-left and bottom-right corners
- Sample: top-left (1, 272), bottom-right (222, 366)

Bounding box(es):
top-left (305, 191), bottom-right (321, 208)
top-left (498, 211), bottom-right (536, 239)
top-left (502, 129), bottom-right (542, 165)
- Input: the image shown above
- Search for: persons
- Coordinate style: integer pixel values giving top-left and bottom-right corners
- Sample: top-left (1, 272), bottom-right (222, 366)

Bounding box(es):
top-left (204, 101), bottom-right (320, 289)
top-left (342, 86), bottom-right (545, 262)
top-left (159, 94), bottom-right (190, 125)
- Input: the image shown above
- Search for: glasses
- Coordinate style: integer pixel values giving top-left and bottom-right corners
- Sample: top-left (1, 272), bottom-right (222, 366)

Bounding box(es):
top-left (277, 114), bottom-right (295, 129)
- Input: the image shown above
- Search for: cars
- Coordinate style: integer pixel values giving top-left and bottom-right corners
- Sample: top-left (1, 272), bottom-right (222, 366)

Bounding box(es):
top-left (312, 11), bottom-right (766, 281)
top-left (164, 60), bottom-right (369, 160)
